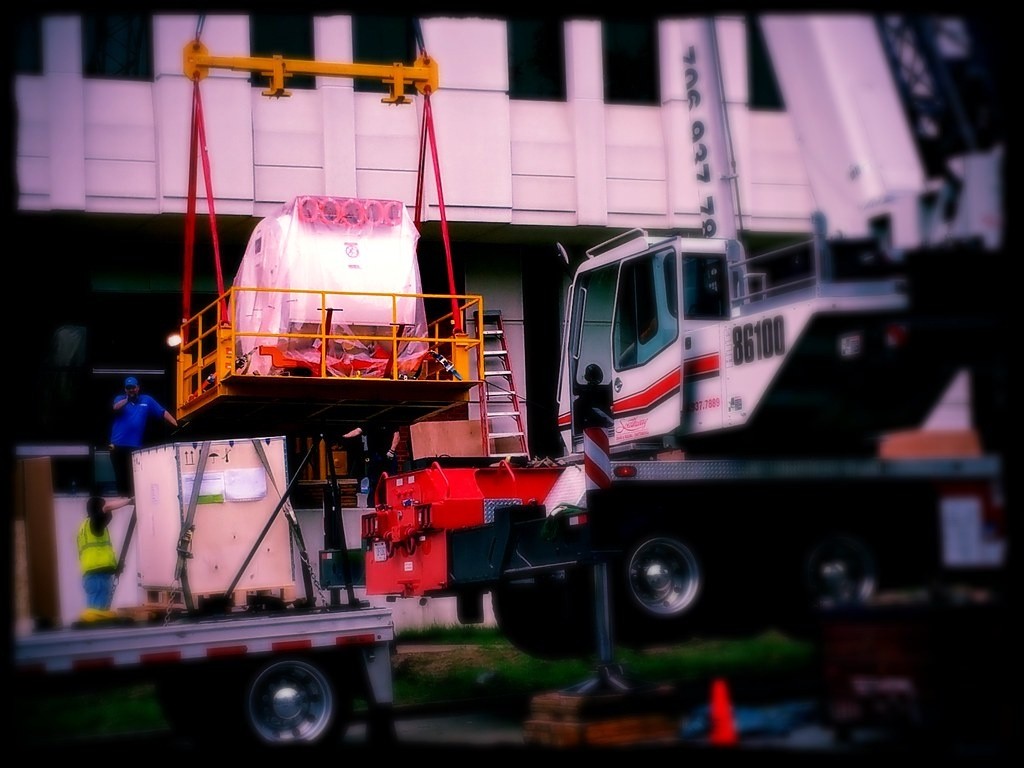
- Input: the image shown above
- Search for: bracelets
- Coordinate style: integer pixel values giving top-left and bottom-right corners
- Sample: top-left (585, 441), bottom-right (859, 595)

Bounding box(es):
top-left (389, 448), bottom-right (395, 454)
top-left (128, 498), bottom-right (134, 505)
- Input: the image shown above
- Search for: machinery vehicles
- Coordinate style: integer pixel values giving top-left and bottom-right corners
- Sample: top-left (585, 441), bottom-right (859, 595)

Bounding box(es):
top-left (365, 11), bottom-right (1018, 645)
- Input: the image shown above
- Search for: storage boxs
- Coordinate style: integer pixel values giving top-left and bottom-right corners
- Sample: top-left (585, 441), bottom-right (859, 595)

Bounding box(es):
top-left (410, 418), bottom-right (495, 461)
top-left (129, 433), bottom-right (296, 593)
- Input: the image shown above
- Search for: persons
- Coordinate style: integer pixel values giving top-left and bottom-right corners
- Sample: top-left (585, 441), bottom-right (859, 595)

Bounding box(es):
top-left (77, 496), bottom-right (136, 609)
top-left (109, 377), bottom-right (178, 495)
top-left (343, 421), bottom-right (400, 508)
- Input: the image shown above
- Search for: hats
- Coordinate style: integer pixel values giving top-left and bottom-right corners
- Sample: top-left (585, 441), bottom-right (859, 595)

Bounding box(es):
top-left (125, 377), bottom-right (139, 387)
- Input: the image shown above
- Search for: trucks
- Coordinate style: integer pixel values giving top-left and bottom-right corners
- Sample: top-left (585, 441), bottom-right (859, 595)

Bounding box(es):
top-left (10, 453), bottom-right (399, 751)
